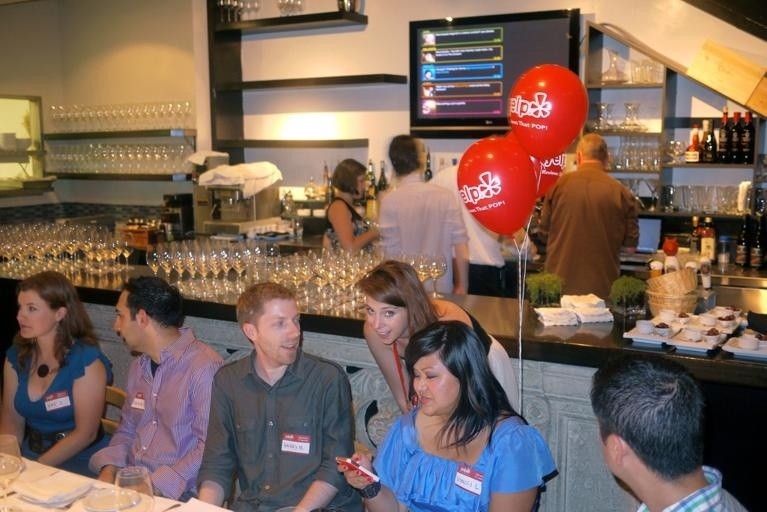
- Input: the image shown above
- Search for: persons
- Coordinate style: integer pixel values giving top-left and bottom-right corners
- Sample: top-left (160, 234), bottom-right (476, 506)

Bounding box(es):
top-left (375, 134), bottom-right (470, 296)
top-left (336, 319), bottom-right (555, 511)
top-left (88, 275), bottom-right (222, 499)
top-left (424, 165), bottom-right (536, 298)
top-left (323, 157), bottom-right (381, 258)
top-left (195, 280), bottom-right (362, 511)
top-left (588, 352), bottom-right (748, 512)
top-left (421, 30), bottom-right (436, 113)
top-left (361, 260), bottom-right (521, 423)
top-left (1, 269), bottom-right (110, 479)
top-left (536, 132), bottom-right (640, 304)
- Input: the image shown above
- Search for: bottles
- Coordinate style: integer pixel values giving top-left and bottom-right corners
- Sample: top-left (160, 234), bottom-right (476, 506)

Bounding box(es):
top-left (280, 190), bottom-right (298, 220)
top-left (689, 212), bottom-right (763, 271)
top-left (164, 221), bottom-right (174, 240)
top-left (304, 146), bottom-right (460, 222)
top-left (597, 47), bottom-right (629, 85)
top-left (586, 101), bottom-right (645, 133)
top-left (683, 104), bottom-right (753, 164)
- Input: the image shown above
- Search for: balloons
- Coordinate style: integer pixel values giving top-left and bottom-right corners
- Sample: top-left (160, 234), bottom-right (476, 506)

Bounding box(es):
top-left (506, 128), bottom-right (565, 198)
top-left (506, 64), bottom-right (589, 162)
top-left (457, 134), bottom-right (537, 239)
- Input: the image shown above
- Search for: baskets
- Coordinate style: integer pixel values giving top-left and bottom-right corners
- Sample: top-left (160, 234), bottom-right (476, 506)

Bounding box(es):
top-left (648, 301), bottom-right (701, 318)
top-left (646, 268), bottom-right (700, 297)
top-left (646, 289), bottom-right (700, 305)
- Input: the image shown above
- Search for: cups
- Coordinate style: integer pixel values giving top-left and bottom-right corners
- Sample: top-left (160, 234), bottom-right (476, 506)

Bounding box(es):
top-left (631, 59), bottom-right (660, 83)
top-left (113, 465), bottom-right (153, 511)
top-left (664, 184), bottom-right (736, 213)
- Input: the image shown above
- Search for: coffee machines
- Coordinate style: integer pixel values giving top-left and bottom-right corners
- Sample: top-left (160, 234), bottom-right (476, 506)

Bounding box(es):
top-left (163, 192), bottom-right (192, 239)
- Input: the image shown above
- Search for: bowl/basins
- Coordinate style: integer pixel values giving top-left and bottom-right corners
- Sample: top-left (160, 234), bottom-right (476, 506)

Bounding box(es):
top-left (635, 308), bottom-right (759, 350)
top-left (0, 131), bottom-right (16, 151)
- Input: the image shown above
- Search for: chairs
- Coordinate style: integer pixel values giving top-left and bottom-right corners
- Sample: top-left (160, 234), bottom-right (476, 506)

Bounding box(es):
top-left (101, 387), bottom-right (129, 434)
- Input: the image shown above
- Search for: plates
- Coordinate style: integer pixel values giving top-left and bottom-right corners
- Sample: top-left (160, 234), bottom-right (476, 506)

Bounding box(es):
top-left (622, 310), bottom-right (767, 359)
top-left (14, 137), bottom-right (33, 151)
top-left (82, 488), bottom-right (140, 512)
top-left (19, 483), bottom-right (92, 504)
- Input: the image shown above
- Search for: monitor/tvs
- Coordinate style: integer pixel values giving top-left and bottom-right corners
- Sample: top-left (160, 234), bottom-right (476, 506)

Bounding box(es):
top-left (406, 6), bottom-right (583, 140)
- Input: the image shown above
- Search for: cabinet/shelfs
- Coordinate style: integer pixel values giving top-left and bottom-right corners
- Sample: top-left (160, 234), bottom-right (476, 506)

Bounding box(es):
top-left (1, 129), bottom-right (198, 198)
top-left (209, 10), bottom-right (409, 152)
top-left (577, 20), bottom-right (762, 225)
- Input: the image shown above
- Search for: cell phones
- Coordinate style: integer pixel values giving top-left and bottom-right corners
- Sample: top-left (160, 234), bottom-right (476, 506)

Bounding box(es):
top-left (333, 453), bottom-right (380, 487)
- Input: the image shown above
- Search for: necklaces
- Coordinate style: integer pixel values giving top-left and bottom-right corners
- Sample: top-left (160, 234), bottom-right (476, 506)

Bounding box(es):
top-left (37, 362), bottom-right (58, 378)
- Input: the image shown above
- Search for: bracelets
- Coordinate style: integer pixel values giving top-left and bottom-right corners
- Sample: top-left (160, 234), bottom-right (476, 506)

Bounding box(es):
top-left (360, 481), bottom-right (381, 499)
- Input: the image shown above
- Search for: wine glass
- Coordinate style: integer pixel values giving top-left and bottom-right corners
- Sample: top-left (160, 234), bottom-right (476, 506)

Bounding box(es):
top-left (45, 98), bottom-right (196, 130)
top-left (217, 0), bottom-right (302, 22)
top-left (0, 433), bottom-right (23, 512)
top-left (144, 234), bottom-right (449, 313)
top-left (618, 177), bottom-right (660, 212)
top-left (0, 223), bottom-right (134, 279)
top-left (617, 133), bottom-right (663, 171)
top-left (47, 143), bottom-right (189, 175)
top-left (665, 140), bottom-right (685, 166)
